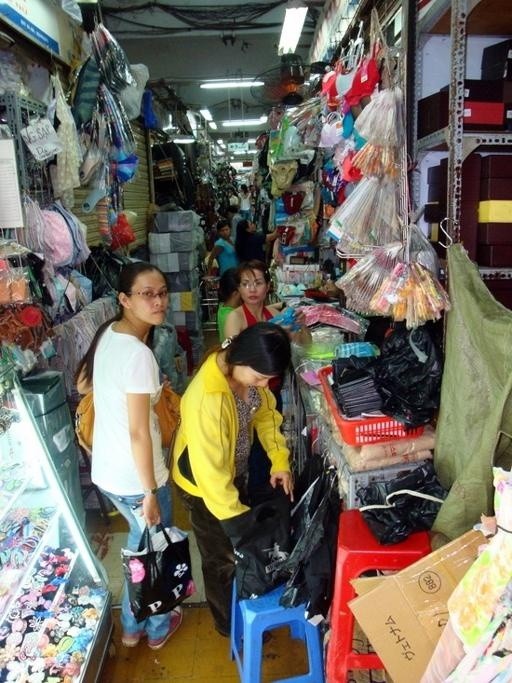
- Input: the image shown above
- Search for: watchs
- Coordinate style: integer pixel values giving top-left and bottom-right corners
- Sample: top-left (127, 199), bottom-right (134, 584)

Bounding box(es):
top-left (144, 488), bottom-right (158, 496)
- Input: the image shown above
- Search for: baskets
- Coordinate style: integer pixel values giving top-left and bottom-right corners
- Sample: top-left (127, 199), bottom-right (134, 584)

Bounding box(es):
top-left (317, 365), bottom-right (424, 447)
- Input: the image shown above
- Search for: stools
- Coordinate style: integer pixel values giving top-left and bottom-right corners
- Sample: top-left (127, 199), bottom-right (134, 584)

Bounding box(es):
top-left (230, 577), bottom-right (324, 683)
top-left (326, 509), bottom-right (431, 683)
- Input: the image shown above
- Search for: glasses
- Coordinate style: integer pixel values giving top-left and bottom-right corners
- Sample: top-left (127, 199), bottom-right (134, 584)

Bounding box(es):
top-left (129, 290), bottom-right (168, 296)
top-left (237, 280), bottom-right (266, 286)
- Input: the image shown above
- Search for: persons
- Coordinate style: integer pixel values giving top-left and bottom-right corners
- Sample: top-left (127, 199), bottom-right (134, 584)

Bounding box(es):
top-left (223, 259), bottom-right (312, 505)
top-left (76, 262), bottom-right (184, 649)
top-left (207, 183), bottom-right (288, 344)
top-left (170, 322), bottom-right (295, 643)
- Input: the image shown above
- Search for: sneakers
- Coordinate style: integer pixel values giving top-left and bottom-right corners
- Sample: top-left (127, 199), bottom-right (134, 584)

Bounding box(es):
top-left (147, 606), bottom-right (183, 649)
top-left (121, 631), bottom-right (145, 649)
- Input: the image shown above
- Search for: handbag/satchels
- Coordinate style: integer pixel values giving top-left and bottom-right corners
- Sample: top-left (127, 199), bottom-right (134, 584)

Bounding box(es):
top-left (236, 486), bottom-right (292, 601)
top-left (74, 385), bottom-right (182, 455)
top-left (121, 526), bottom-right (197, 623)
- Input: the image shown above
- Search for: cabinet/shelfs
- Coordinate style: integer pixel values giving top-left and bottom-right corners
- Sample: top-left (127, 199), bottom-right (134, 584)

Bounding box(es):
top-left (413, 0), bottom-right (512, 362)
top-left (0, 366), bottom-right (115, 683)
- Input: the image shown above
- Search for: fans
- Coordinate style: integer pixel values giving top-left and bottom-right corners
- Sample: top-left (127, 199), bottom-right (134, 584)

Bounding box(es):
top-left (250, 53), bottom-right (326, 108)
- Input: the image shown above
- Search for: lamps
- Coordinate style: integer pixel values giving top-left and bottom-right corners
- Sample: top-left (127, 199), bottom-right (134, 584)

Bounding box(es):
top-left (278, 1), bottom-right (309, 57)
